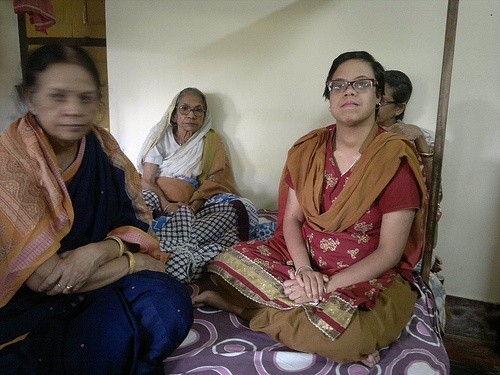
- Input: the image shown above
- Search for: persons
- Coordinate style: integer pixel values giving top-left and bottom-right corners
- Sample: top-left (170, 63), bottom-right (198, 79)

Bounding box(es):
top-left (191, 50), bottom-right (430, 367)
top-left (376, 69), bottom-right (443, 274)
top-left (0, 44), bottom-right (195, 375)
top-left (133, 87), bottom-right (259, 284)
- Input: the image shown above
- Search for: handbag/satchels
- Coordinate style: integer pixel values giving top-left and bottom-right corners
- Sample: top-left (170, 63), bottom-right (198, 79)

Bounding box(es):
top-left (0, 293), bottom-right (85, 375)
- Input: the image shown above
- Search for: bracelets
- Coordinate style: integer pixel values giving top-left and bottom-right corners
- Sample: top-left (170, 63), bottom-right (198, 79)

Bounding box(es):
top-left (104, 235), bottom-right (124, 258)
top-left (323, 285), bottom-right (327, 294)
top-left (295, 265), bottom-right (313, 276)
top-left (420, 150), bottom-right (433, 157)
top-left (124, 251), bottom-right (136, 275)
top-left (163, 203), bottom-right (171, 212)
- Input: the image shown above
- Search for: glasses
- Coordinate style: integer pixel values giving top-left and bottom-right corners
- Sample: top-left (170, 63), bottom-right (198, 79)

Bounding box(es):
top-left (379, 98), bottom-right (398, 107)
top-left (326, 78), bottom-right (377, 93)
top-left (176, 103), bottom-right (207, 116)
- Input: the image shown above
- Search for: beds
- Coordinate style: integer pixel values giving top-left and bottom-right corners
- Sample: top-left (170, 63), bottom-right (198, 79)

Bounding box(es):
top-left (161, 208), bottom-right (450, 375)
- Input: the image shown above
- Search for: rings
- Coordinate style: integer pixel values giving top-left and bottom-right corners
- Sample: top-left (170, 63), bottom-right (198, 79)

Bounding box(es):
top-left (56, 283), bottom-right (65, 290)
top-left (66, 285), bottom-right (74, 292)
top-left (289, 286), bottom-right (293, 293)
